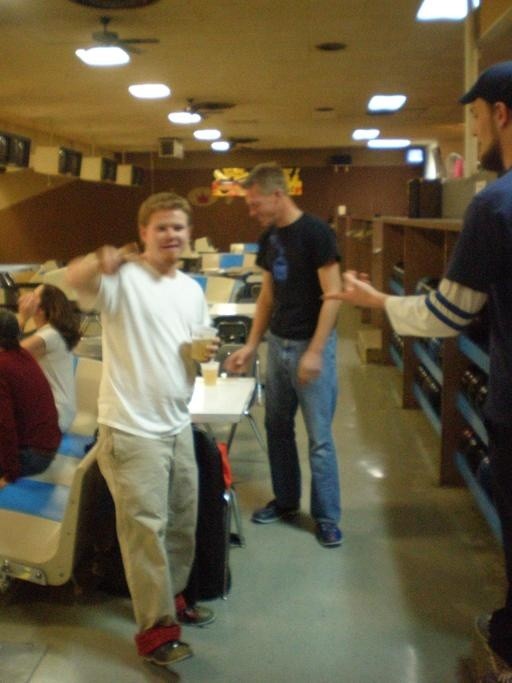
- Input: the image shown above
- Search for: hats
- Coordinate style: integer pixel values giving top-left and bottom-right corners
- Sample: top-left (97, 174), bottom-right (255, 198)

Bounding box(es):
top-left (460, 61), bottom-right (512, 103)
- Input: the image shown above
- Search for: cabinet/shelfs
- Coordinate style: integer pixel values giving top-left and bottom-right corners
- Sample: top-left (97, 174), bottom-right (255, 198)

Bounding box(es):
top-left (465, 1), bottom-right (511, 181)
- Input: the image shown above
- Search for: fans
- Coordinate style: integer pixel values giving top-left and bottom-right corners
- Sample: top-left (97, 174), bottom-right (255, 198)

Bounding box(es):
top-left (75, 14), bottom-right (259, 151)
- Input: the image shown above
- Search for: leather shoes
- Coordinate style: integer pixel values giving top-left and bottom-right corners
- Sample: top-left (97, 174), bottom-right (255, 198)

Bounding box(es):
top-left (145, 640), bottom-right (192, 665)
top-left (179, 605), bottom-right (215, 625)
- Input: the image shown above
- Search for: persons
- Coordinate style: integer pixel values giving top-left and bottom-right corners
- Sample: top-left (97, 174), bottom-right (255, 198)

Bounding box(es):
top-left (321, 62), bottom-right (511, 662)
top-left (16, 283), bottom-right (83, 434)
top-left (62, 192), bottom-right (222, 668)
top-left (0, 310), bottom-right (65, 491)
top-left (222, 161), bottom-right (342, 547)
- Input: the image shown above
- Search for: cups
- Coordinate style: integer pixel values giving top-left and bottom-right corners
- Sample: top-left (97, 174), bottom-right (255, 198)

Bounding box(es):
top-left (189, 323), bottom-right (220, 363)
top-left (199, 361), bottom-right (220, 387)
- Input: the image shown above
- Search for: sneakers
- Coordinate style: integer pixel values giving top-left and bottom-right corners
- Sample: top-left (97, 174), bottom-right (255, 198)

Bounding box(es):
top-left (317, 523), bottom-right (343, 545)
top-left (253, 501), bottom-right (299, 522)
